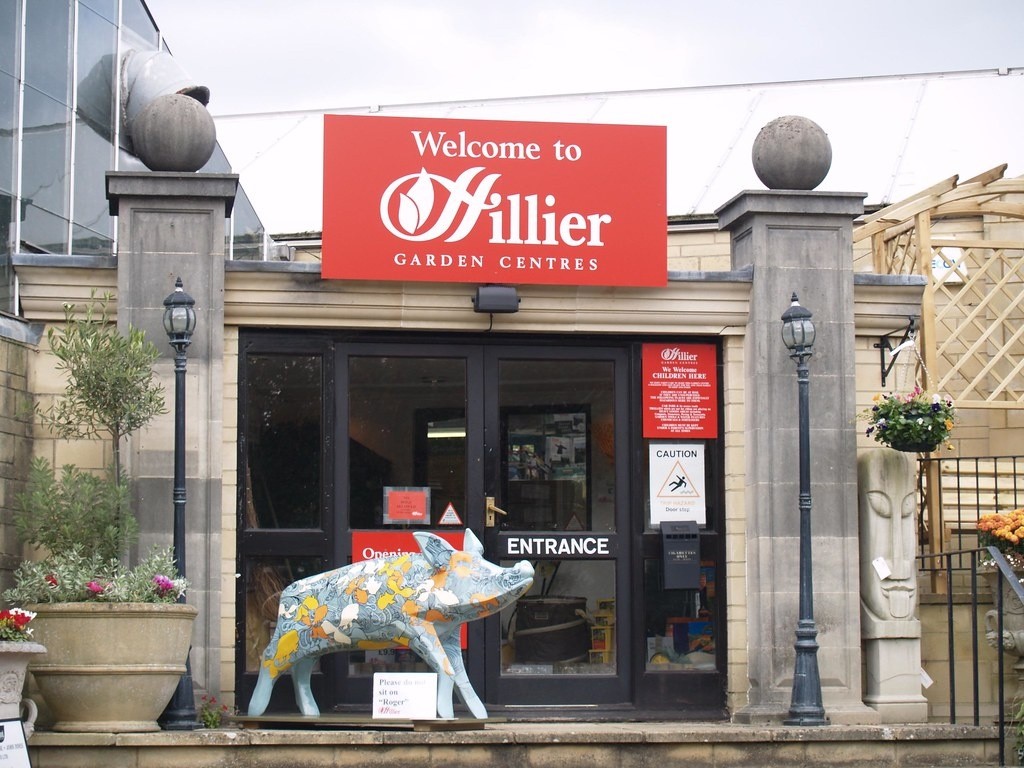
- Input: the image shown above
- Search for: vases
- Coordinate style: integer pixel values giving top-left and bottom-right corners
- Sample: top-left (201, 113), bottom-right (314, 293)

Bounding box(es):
top-left (975, 559), bottom-right (1024, 723)
top-left (0, 641), bottom-right (48, 721)
top-left (20, 603), bottom-right (198, 732)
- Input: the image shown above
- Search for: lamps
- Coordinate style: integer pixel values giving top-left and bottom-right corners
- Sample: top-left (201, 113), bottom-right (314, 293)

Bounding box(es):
top-left (780, 292), bottom-right (818, 369)
top-left (163, 276), bottom-right (197, 354)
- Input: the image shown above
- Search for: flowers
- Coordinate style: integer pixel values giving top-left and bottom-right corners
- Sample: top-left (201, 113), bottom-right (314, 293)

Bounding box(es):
top-left (974, 508), bottom-right (1024, 560)
top-left (1, 545), bottom-right (191, 608)
top-left (0, 607), bottom-right (38, 642)
top-left (846, 385), bottom-right (957, 452)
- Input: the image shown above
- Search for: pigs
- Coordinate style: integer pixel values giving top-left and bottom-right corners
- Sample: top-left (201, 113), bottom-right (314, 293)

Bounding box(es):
top-left (247, 529), bottom-right (535, 720)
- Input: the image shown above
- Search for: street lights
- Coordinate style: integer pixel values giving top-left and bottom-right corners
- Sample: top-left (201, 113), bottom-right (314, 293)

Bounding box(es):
top-left (162, 277), bottom-right (204, 732)
top-left (781, 291), bottom-right (831, 726)
